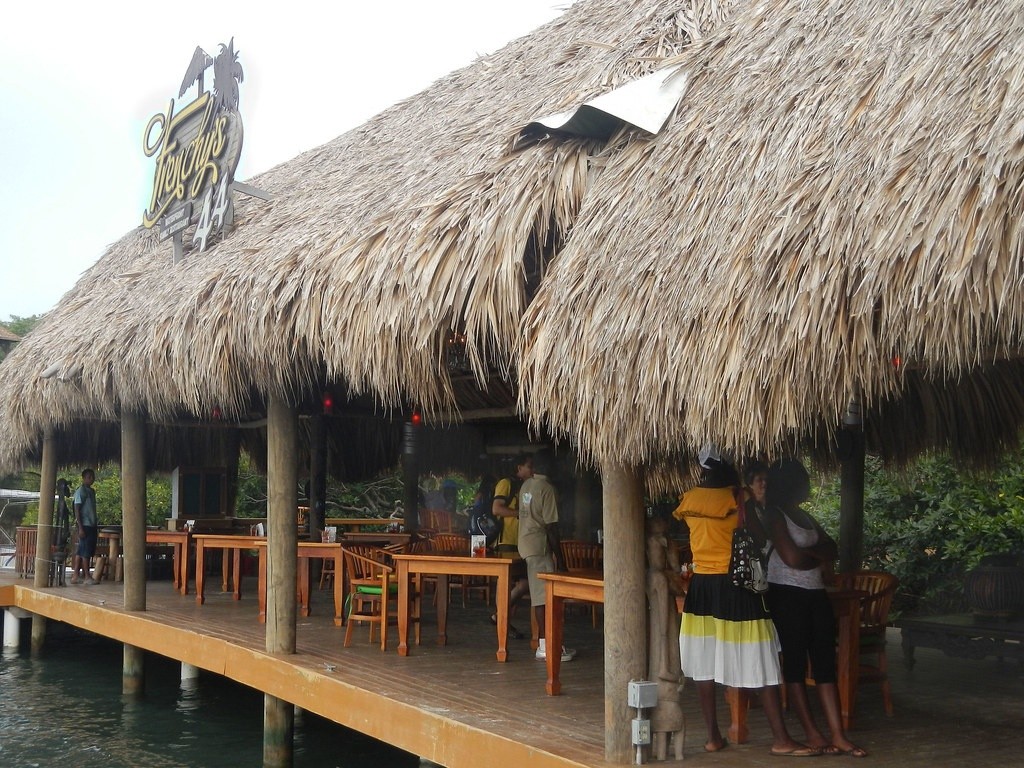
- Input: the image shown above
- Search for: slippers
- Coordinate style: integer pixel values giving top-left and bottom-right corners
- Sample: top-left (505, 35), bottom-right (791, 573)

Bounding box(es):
top-left (822, 744), bottom-right (866, 757)
top-left (704, 736), bottom-right (726, 752)
top-left (771, 747), bottom-right (823, 756)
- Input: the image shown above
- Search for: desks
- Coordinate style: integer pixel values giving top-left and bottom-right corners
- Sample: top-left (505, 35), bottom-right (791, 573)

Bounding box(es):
top-left (343, 532), bottom-right (412, 554)
top-left (536, 569), bottom-right (870, 746)
top-left (392, 552), bottom-right (539, 662)
top-left (254, 540), bottom-right (364, 627)
top-left (899, 612), bottom-right (1024, 671)
top-left (191, 534), bottom-right (308, 604)
top-left (144, 532), bottom-right (231, 594)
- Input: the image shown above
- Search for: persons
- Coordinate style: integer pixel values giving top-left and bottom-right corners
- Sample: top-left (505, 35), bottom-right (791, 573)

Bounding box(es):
top-left (470, 450), bottom-right (576, 663)
top-left (672, 445), bottom-right (822, 758)
top-left (70, 469), bottom-right (101, 585)
top-left (742, 467), bottom-right (772, 556)
top-left (759, 457), bottom-right (868, 758)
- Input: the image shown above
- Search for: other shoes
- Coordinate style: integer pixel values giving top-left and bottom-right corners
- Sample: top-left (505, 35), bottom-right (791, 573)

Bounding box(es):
top-left (490, 614), bottom-right (522, 639)
top-left (85, 579), bottom-right (99, 585)
top-left (536, 644), bottom-right (576, 661)
top-left (71, 579), bottom-right (83, 584)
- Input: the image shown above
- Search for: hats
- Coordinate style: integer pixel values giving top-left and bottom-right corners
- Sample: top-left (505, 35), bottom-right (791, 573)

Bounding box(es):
top-left (698, 442), bottom-right (734, 469)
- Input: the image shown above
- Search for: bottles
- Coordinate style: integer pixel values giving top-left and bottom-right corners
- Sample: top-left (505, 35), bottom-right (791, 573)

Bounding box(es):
top-left (184, 522), bottom-right (188, 532)
top-left (385, 524), bottom-right (401, 534)
top-left (189, 525), bottom-right (195, 532)
top-left (325, 524), bottom-right (329, 527)
top-left (251, 524), bottom-right (256, 536)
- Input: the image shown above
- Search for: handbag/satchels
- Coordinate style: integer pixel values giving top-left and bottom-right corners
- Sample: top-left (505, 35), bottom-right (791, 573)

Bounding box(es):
top-left (728, 528), bottom-right (769, 593)
top-left (469, 505), bottom-right (502, 546)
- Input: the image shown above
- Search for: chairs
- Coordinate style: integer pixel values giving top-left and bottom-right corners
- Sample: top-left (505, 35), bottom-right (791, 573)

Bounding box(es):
top-left (784, 570), bottom-right (899, 719)
top-left (78, 509), bottom-right (604, 651)
